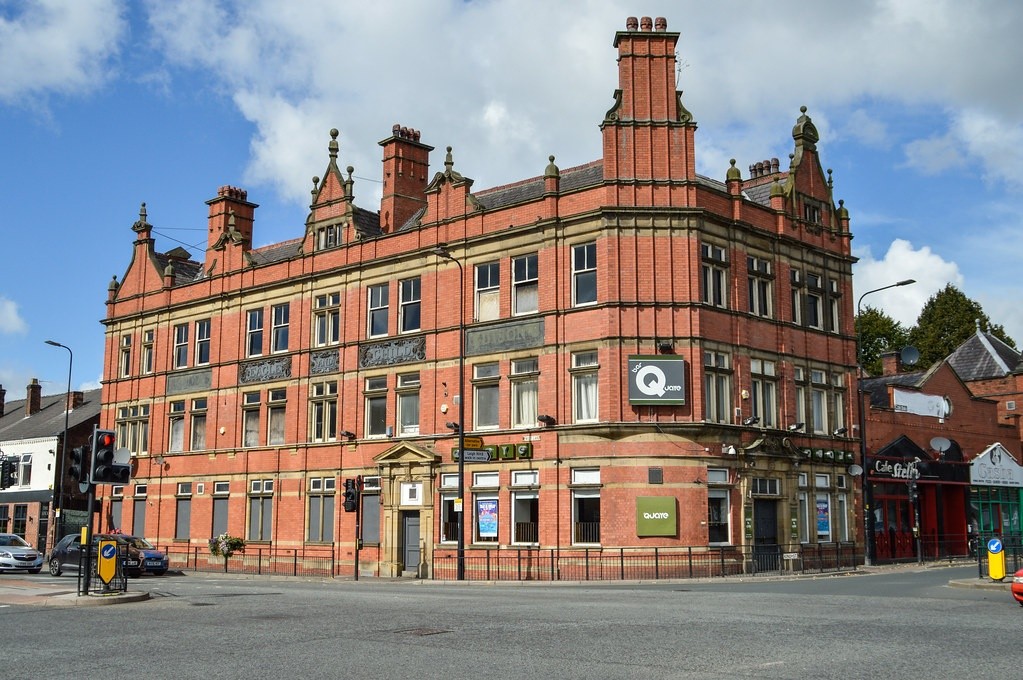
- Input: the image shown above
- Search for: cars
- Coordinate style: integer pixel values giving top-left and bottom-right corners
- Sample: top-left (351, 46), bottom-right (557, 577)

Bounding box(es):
top-left (0, 534), bottom-right (43, 575)
top-left (1011, 569), bottom-right (1023, 604)
top-left (119, 534), bottom-right (169, 577)
top-left (47, 534), bottom-right (147, 578)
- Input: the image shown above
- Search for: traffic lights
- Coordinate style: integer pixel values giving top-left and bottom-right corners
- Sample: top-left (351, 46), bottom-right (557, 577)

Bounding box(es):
top-left (342, 479), bottom-right (357, 512)
top-left (1, 460), bottom-right (16, 489)
top-left (91, 429), bottom-right (131, 486)
top-left (69, 446), bottom-right (87, 484)
top-left (909, 481), bottom-right (919, 502)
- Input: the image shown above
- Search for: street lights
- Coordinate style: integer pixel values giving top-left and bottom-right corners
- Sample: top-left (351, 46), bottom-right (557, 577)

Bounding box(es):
top-left (44, 340), bottom-right (72, 543)
top-left (437, 250), bottom-right (465, 581)
top-left (856, 278), bottom-right (917, 567)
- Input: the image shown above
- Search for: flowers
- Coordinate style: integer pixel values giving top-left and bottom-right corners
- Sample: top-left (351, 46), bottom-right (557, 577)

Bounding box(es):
top-left (208, 532), bottom-right (247, 557)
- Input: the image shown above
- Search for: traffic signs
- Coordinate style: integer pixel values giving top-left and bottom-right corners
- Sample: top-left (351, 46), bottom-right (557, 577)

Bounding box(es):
top-left (462, 436), bottom-right (491, 462)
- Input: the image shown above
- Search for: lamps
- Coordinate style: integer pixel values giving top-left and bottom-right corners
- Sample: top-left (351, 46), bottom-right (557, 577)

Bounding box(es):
top-left (832, 427), bottom-right (848, 436)
top-left (788, 423), bottom-right (804, 430)
top-left (658, 343), bottom-right (671, 351)
top-left (538, 415), bottom-right (555, 424)
top-left (340, 430), bottom-right (355, 439)
top-left (743, 416), bottom-right (760, 426)
top-left (446, 421), bottom-right (460, 432)
